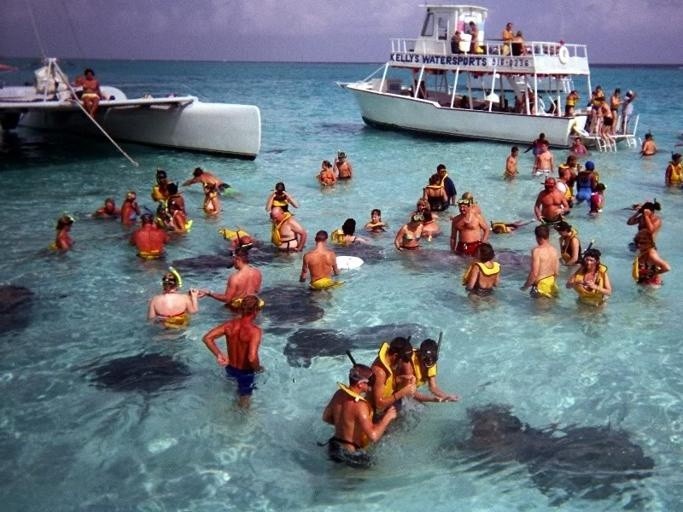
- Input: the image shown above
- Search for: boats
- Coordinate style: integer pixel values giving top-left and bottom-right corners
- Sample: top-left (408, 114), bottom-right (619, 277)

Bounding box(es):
top-left (331, 2), bottom-right (642, 152)
top-left (0, 83), bottom-right (263, 163)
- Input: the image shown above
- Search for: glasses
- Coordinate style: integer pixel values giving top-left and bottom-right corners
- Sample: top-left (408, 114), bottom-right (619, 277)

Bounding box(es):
top-left (365, 375), bottom-right (378, 387)
top-left (419, 354), bottom-right (440, 365)
top-left (413, 215), bottom-right (424, 222)
top-left (553, 222), bottom-right (565, 230)
top-left (457, 199), bottom-right (470, 204)
top-left (398, 350), bottom-right (415, 363)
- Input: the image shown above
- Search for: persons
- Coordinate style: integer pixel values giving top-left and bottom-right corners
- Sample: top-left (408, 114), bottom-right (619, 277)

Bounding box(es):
top-left (404, 339), bottom-right (460, 405)
top-left (642, 132), bottom-right (656, 155)
top-left (590, 85), bottom-right (636, 144)
top-left (220, 228), bottom-right (254, 251)
top-left (55, 215), bottom-right (76, 252)
top-left (73, 68), bottom-right (105, 120)
top-left (409, 79), bottom-right (427, 99)
top-left (483, 89), bottom-right (557, 115)
top-left (336, 209), bottom-right (385, 243)
top-left (265, 182), bottom-right (307, 251)
top-left (320, 363), bottom-right (399, 469)
top-left (506, 133), bottom-right (612, 306)
top-left (148, 248), bottom-right (261, 329)
top-left (443, 95), bottom-right (470, 109)
top-left (93, 167), bottom-right (227, 261)
top-left (301, 230), bottom-right (338, 288)
top-left (319, 152), bottom-right (352, 186)
top-left (364, 336), bottom-right (418, 420)
top-left (666, 153), bottom-right (683, 187)
top-left (565, 90), bottom-right (580, 116)
top-left (452, 21), bottom-right (564, 55)
top-left (200, 295), bottom-right (264, 409)
top-left (627, 201), bottom-right (671, 286)
top-left (395, 164), bottom-right (501, 296)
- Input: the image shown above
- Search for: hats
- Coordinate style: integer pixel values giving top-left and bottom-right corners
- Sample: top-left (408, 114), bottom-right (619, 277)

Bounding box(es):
top-left (541, 177), bottom-right (557, 187)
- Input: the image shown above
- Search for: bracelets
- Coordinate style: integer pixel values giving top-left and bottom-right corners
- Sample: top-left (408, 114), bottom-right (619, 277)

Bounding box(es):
top-left (392, 392), bottom-right (397, 403)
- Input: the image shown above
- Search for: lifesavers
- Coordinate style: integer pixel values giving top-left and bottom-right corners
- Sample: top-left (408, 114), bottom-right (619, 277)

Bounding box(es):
top-left (559, 46), bottom-right (569, 64)
top-left (336, 256), bottom-right (364, 270)
